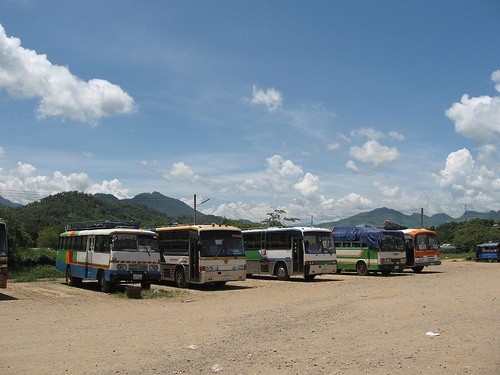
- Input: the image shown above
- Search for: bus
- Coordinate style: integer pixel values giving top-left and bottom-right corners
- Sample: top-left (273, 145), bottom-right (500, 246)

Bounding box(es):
top-left (239, 226), bottom-right (338, 281)
top-left (0, 218), bottom-right (8, 289)
top-left (143, 223), bottom-right (247, 287)
top-left (476, 242), bottom-right (499, 262)
top-left (399, 229), bottom-right (442, 272)
top-left (56, 228), bottom-right (162, 292)
top-left (329, 225), bottom-right (406, 276)
